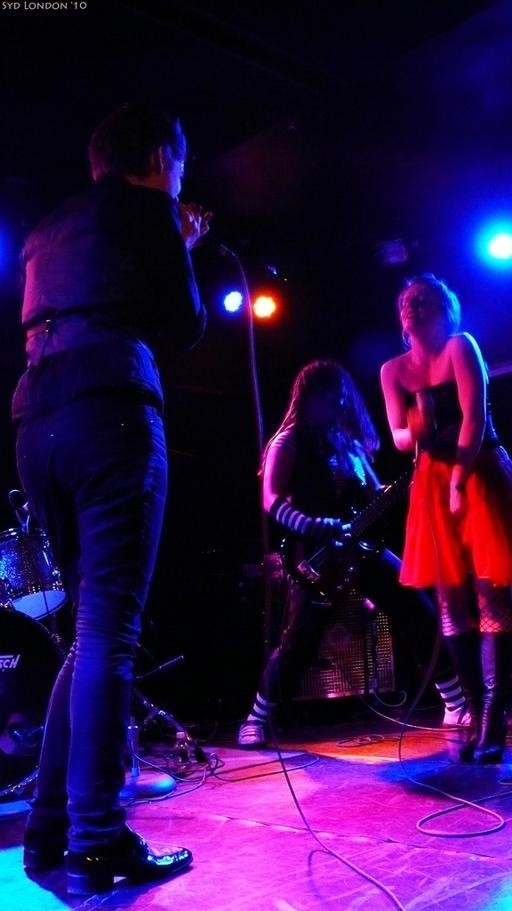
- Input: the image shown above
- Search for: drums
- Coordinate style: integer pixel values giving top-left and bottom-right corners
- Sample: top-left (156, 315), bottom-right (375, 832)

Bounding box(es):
top-left (0, 604), bottom-right (67, 803)
top-left (0, 528), bottom-right (69, 621)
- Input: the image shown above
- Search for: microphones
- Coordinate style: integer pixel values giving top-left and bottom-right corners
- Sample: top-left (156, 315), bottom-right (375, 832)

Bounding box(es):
top-left (204, 234), bottom-right (238, 259)
top-left (416, 392), bottom-right (435, 453)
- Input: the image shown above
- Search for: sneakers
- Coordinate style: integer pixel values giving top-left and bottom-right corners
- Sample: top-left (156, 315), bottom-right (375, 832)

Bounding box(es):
top-left (238, 719), bottom-right (266, 746)
top-left (443, 702), bottom-right (477, 726)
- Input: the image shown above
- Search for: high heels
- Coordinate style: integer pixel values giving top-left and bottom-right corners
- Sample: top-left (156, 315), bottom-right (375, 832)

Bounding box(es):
top-left (470, 630), bottom-right (505, 760)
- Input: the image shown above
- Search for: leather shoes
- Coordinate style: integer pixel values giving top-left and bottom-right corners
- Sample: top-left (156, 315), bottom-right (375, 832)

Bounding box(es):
top-left (24, 824), bottom-right (192, 894)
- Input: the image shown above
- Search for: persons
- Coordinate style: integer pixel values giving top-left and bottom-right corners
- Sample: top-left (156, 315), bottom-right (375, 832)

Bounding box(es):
top-left (8, 101), bottom-right (213, 894)
top-left (238, 358), bottom-right (476, 748)
top-left (378, 273), bottom-right (511, 761)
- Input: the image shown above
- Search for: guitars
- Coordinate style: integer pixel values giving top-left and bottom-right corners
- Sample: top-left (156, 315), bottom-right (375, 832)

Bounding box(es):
top-left (274, 468), bottom-right (411, 602)
